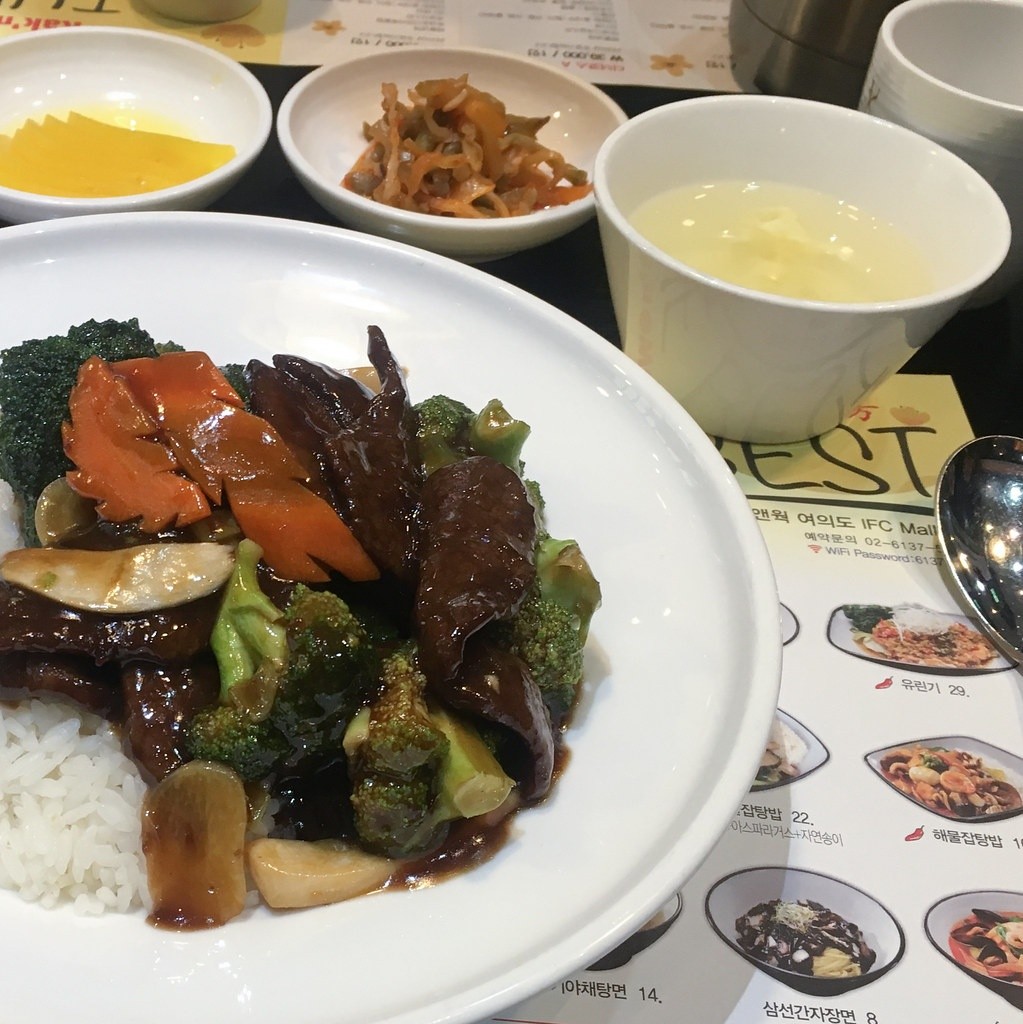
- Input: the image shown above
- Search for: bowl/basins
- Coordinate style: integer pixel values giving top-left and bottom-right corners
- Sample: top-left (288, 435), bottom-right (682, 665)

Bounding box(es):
top-left (924, 889), bottom-right (1023, 1012)
top-left (592, 95), bottom-right (1012, 446)
top-left (704, 865), bottom-right (906, 998)
top-left (582, 891), bottom-right (682, 972)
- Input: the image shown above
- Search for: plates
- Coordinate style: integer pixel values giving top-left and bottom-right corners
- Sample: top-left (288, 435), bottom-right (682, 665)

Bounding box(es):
top-left (0, 211), bottom-right (785, 1024)
top-left (824, 601), bottom-right (1017, 675)
top-left (863, 735), bottom-right (1023, 825)
top-left (0, 26), bottom-right (272, 223)
top-left (748, 707), bottom-right (831, 792)
top-left (277, 47), bottom-right (633, 264)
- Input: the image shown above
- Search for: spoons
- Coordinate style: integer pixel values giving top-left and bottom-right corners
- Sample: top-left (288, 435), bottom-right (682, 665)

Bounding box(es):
top-left (935, 435), bottom-right (1023, 678)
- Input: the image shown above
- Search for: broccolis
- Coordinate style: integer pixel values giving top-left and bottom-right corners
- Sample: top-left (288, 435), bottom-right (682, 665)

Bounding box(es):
top-left (0, 320), bottom-right (160, 553)
top-left (192, 542), bottom-right (380, 780)
top-left (515, 539), bottom-right (599, 716)
top-left (415, 396), bottom-right (546, 537)
top-left (348, 654), bottom-right (510, 856)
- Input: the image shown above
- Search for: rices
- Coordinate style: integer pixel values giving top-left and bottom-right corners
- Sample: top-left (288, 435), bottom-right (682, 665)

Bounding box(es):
top-left (0, 686), bottom-right (152, 915)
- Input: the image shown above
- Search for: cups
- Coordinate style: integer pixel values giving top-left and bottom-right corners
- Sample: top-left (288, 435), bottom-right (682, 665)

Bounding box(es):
top-left (857, 0), bottom-right (1023, 200)
